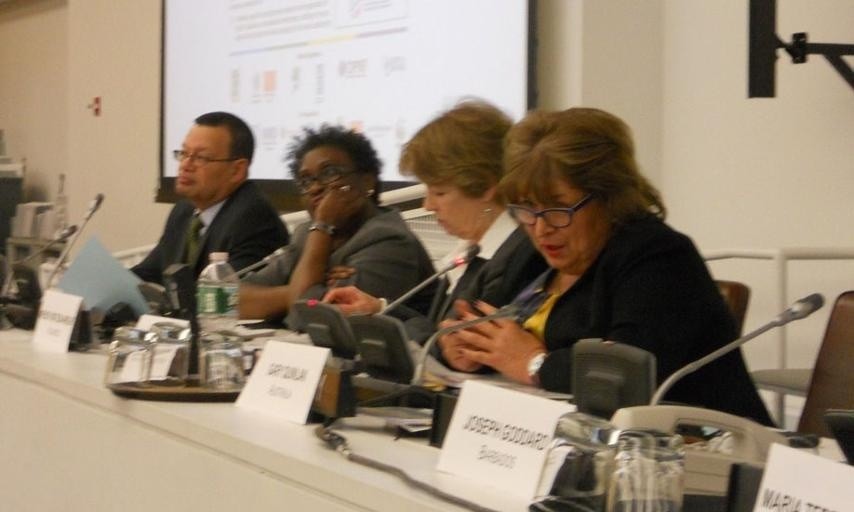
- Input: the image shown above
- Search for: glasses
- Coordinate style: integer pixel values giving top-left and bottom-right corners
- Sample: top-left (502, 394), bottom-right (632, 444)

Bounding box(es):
top-left (294, 162), bottom-right (358, 195)
top-left (504, 192), bottom-right (595, 227)
top-left (173, 150), bottom-right (236, 167)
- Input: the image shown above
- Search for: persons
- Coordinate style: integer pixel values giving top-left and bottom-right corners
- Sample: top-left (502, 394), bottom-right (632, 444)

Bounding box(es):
top-left (435, 108), bottom-right (776, 428)
top-left (237, 124), bottom-right (446, 333)
top-left (127, 111), bottom-right (291, 330)
top-left (319, 96), bottom-right (549, 372)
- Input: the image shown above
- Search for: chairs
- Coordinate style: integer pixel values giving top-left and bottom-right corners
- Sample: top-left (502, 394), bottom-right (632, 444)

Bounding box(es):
top-left (716, 280), bottom-right (751, 335)
top-left (799, 290), bottom-right (854, 438)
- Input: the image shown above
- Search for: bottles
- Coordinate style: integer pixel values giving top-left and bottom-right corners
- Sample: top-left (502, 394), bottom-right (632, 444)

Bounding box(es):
top-left (195, 248), bottom-right (242, 385)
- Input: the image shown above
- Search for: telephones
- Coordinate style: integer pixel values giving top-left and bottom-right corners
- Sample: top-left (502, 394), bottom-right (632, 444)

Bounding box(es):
top-left (555, 406), bottom-right (790, 511)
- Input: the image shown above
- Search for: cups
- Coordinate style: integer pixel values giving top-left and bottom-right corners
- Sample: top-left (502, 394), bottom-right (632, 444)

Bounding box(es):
top-left (104, 321), bottom-right (192, 388)
top-left (531, 413), bottom-right (687, 512)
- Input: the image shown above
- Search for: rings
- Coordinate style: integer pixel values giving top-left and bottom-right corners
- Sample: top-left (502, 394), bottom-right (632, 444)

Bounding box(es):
top-left (339, 184), bottom-right (350, 192)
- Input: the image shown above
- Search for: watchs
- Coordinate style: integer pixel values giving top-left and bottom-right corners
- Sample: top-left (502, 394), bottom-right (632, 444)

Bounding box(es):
top-left (306, 222), bottom-right (340, 240)
top-left (525, 354), bottom-right (547, 381)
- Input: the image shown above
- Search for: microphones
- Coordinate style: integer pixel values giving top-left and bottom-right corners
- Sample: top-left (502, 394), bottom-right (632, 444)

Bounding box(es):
top-left (0, 225), bottom-right (77, 303)
top-left (221, 245), bottom-right (294, 280)
top-left (358, 303), bottom-right (525, 406)
top-left (47, 194), bottom-right (104, 287)
top-left (650, 291), bottom-right (823, 405)
top-left (368, 245), bottom-right (478, 314)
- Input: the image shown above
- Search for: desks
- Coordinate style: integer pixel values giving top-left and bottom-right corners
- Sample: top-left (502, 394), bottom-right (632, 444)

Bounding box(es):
top-left (0, 326), bottom-right (483, 512)
